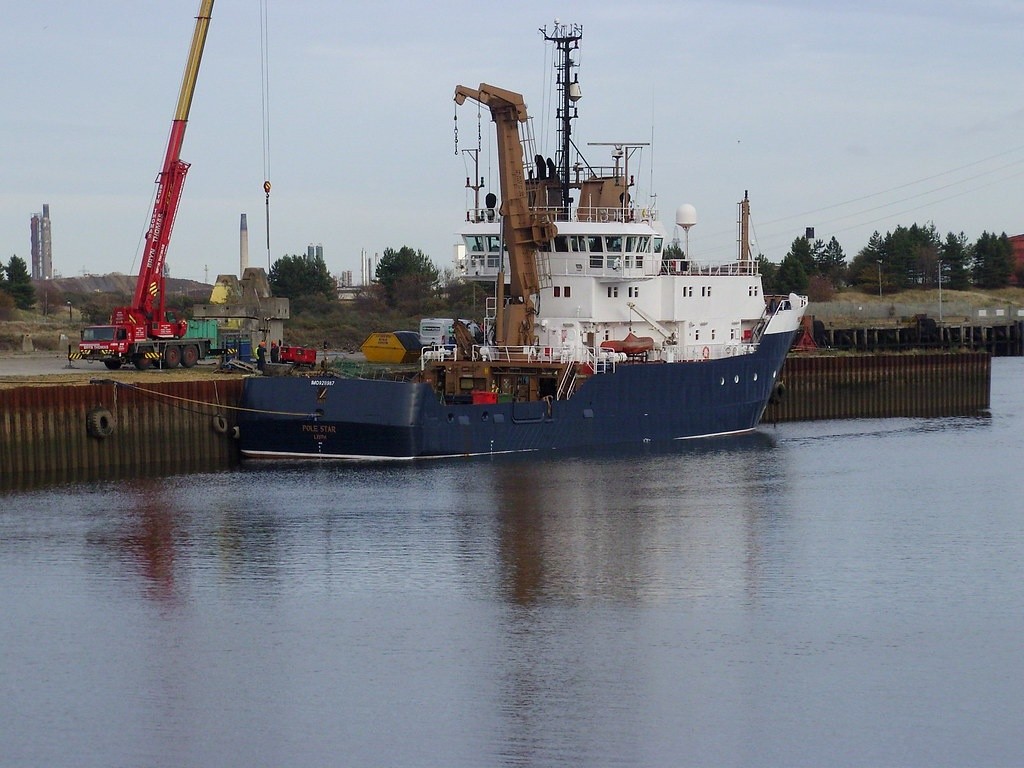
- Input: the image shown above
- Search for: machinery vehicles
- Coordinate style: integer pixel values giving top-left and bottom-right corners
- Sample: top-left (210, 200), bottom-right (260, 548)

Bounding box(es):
top-left (450, 82), bottom-right (560, 359)
top-left (73, 2), bottom-right (213, 370)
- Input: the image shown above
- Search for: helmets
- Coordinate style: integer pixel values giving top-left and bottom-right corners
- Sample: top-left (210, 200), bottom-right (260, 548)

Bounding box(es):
top-left (260, 342), bottom-right (266, 347)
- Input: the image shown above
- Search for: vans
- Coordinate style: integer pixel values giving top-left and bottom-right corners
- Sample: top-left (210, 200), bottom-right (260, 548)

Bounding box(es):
top-left (419, 318), bottom-right (483, 347)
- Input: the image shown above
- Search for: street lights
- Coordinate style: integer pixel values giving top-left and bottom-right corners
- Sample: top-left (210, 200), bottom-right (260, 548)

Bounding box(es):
top-left (877, 259), bottom-right (887, 303)
top-left (937, 259), bottom-right (944, 322)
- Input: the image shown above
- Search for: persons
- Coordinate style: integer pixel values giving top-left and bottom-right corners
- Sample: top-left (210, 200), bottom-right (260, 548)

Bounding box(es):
top-left (254, 340), bottom-right (279, 371)
top-left (491, 378), bottom-right (512, 394)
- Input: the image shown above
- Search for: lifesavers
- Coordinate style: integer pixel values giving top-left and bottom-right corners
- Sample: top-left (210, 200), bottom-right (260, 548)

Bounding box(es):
top-left (88, 408), bottom-right (115, 439)
top-left (703, 346), bottom-right (709, 358)
top-left (213, 414), bottom-right (228, 433)
top-left (773, 381), bottom-right (786, 403)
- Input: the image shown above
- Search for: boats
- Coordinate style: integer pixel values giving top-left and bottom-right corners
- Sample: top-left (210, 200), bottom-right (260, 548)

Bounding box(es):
top-left (602, 332), bottom-right (655, 355)
top-left (226, 19), bottom-right (808, 479)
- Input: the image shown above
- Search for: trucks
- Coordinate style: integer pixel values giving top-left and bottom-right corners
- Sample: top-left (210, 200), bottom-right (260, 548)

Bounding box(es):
top-left (279, 346), bottom-right (317, 368)
